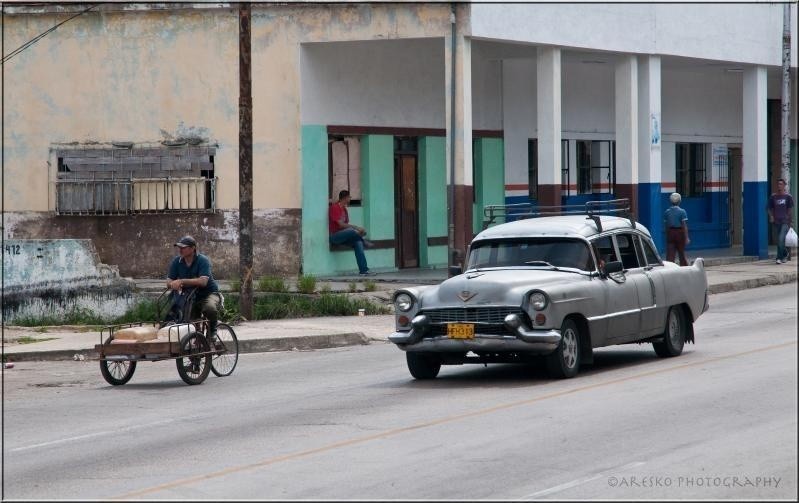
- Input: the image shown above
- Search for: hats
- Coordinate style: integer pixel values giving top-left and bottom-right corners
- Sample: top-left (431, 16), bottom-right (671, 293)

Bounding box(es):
top-left (173, 235), bottom-right (196, 250)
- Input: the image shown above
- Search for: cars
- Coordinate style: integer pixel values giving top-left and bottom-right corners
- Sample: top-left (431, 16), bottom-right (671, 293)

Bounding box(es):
top-left (389, 198), bottom-right (714, 380)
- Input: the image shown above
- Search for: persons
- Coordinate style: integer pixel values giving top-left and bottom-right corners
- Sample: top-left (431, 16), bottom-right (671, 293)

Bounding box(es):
top-left (328, 190), bottom-right (376, 277)
top-left (767, 178), bottom-right (794, 264)
top-left (166, 236), bottom-right (224, 374)
top-left (569, 242), bottom-right (604, 273)
top-left (664, 192), bottom-right (690, 266)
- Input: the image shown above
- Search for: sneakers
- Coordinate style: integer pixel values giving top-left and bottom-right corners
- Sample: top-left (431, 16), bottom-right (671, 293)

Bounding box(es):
top-left (776, 258), bottom-right (781, 264)
top-left (782, 252), bottom-right (789, 263)
top-left (358, 271), bottom-right (377, 276)
top-left (209, 338), bottom-right (217, 354)
top-left (183, 363), bottom-right (201, 375)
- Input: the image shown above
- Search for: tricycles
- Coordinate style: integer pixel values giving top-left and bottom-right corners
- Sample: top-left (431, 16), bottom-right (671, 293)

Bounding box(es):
top-left (88, 283), bottom-right (244, 389)
